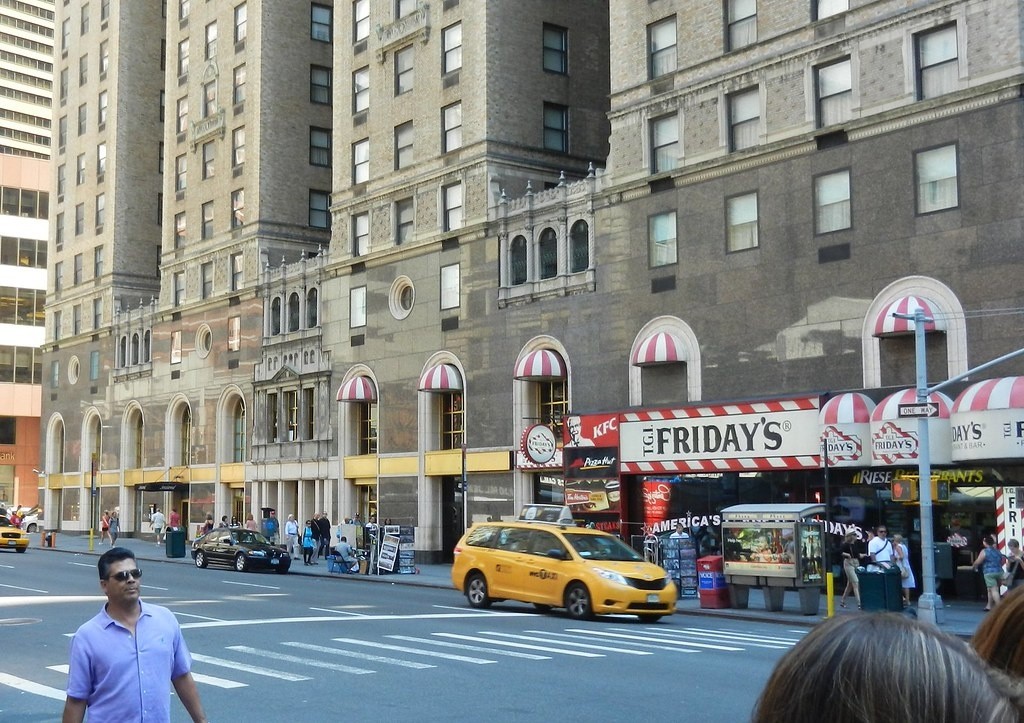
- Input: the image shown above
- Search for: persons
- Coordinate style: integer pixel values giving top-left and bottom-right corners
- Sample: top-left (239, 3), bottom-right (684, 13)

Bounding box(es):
top-left (98, 510), bottom-right (121, 548)
top-left (265, 510), bottom-right (331, 566)
top-left (149, 508), bottom-right (180, 545)
top-left (62, 547), bottom-right (207, 723)
top-left (972, 536), bottom-right (1024, 611)
top-left (840, 525), bottom-right (916, 611)
top-left (202, 514), bottom-right (256, 533)
top-left (0, 504), bottom-right (24, 528)
top-left (668, 523), bottom-right (689, 538)
top-left (751, 582), bottom-right (1024, 723)
top-left (750, 536), bottom-right (794, 562)
top-left (335, 512), bottom-right (391, 574)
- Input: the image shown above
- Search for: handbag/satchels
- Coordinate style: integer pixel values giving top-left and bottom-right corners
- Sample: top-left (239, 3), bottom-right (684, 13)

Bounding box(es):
top-left (1002, 572), bottom-right (1014, 586)
top-left (856, 566), bottom-right (865, 572)
top-left (102, 526), bottom-right (108, 531)
top-left (298, 536), bottom-right (303, 544)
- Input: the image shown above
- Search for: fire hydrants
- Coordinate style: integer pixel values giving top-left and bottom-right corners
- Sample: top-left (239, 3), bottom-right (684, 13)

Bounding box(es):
top-left (45, 532), bottom-right (52, 548)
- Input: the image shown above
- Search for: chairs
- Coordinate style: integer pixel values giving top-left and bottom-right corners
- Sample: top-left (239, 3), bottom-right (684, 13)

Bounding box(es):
top-left (330, 550), bottom-right (352, 574)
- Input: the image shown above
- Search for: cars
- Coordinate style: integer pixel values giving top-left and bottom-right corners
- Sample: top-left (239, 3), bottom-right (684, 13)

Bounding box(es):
top-left (0, 515), bottom-right (30, 553)
top-left (450, 503), bottom-right (678, 624)
top-left (191, 527), bottom-right (291, 575)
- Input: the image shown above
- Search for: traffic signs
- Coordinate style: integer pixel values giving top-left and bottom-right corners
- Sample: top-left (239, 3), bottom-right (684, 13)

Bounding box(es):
top-left (897, 401), bottom-right (940, 418)
top-left (891, 479), bottom-right (913, 502)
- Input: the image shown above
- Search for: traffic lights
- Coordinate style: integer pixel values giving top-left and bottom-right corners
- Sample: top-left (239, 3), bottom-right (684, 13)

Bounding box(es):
top-left (930, 479), bottom-right (951, 502)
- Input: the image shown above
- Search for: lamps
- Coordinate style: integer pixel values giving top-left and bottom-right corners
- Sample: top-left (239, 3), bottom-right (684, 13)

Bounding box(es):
top-left (32, 469), bottom-right (44, 474)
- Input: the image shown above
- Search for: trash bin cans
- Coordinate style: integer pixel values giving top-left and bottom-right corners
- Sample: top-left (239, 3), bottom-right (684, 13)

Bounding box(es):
top-left (933, 542), bottom-right (953, 578)
top-left (855, 561), bottom-right (904, 613)
top-left (165, 526), bottom-right (186, 558)
top-left (697, 556), bottom-right (731, 609)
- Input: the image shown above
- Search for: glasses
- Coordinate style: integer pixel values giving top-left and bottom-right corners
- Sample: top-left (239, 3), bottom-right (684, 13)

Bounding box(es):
top-left (109, 569), bottom-right (143, 581)
top-left (879, 531), bottom-right (887, 534)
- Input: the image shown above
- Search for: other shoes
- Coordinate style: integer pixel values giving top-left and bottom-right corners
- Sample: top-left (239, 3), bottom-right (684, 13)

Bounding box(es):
top-left (306, 561), bottom-right (312, 565)
top-left (294, 557), bottom-right (300, 560)
top-left (99, 543), bottom-right (103, 545)
top-left (304, 564), bottom-right (308, 565)
top-left (113, 544), bottom-right (115, 548)
top-left (840, 602), bottom-right (848, 607)
top-left (982, 607), bottom-right (990, 611)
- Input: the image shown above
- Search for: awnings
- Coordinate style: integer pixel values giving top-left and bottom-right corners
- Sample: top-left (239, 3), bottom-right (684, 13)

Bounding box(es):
top-left (632, 332), bottom-right (687, 368)
top-left (336, 376), bottom-right (377, 403)
top-left (871, 296), bottom-right (947, 340)
top-left (416, 364), bottom-right (462, 393)
top-left (513, 350), bottom-right (567, 383)
